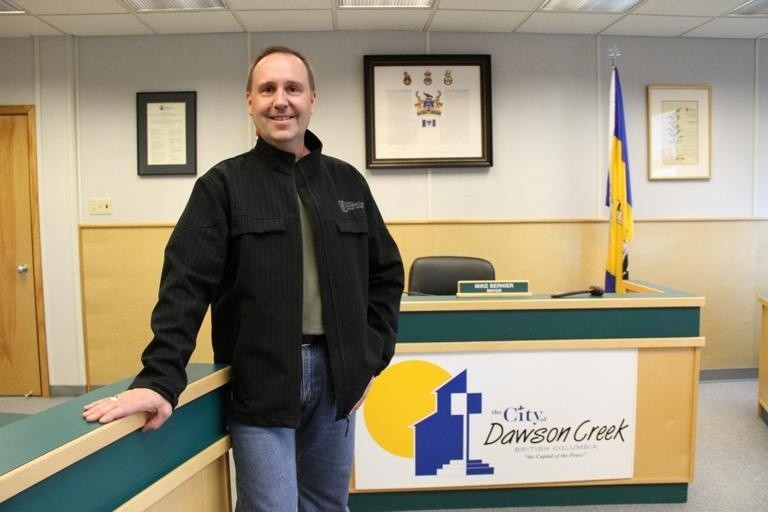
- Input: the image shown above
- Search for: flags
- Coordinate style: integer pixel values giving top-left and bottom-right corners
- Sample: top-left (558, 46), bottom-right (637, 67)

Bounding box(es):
top-left (605, 66), bottom-right (634, 294)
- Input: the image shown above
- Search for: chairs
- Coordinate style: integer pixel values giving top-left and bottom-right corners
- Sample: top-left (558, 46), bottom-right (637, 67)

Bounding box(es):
top-left (408, 256), bottom-right (495, 296)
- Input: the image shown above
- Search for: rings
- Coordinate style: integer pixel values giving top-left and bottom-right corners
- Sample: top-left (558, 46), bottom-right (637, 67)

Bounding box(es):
top-left (111, 396), bottom-right (119, 401)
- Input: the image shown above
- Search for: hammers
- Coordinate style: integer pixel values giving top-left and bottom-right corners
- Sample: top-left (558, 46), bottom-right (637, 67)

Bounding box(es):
top-left (551, 285), bottom-right (605, 298)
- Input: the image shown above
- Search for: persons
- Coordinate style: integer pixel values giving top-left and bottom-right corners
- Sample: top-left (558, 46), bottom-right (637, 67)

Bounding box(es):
top-left (82, 47), bottom-right (405, 512)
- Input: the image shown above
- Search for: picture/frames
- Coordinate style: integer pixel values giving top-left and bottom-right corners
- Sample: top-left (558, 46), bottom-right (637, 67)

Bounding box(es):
top-left (363, 54), bottom-right (492, 168)
top-left (646, 84), bottom-right (711, 179)
top-left (136, 90), bottom-right (197, 175)
